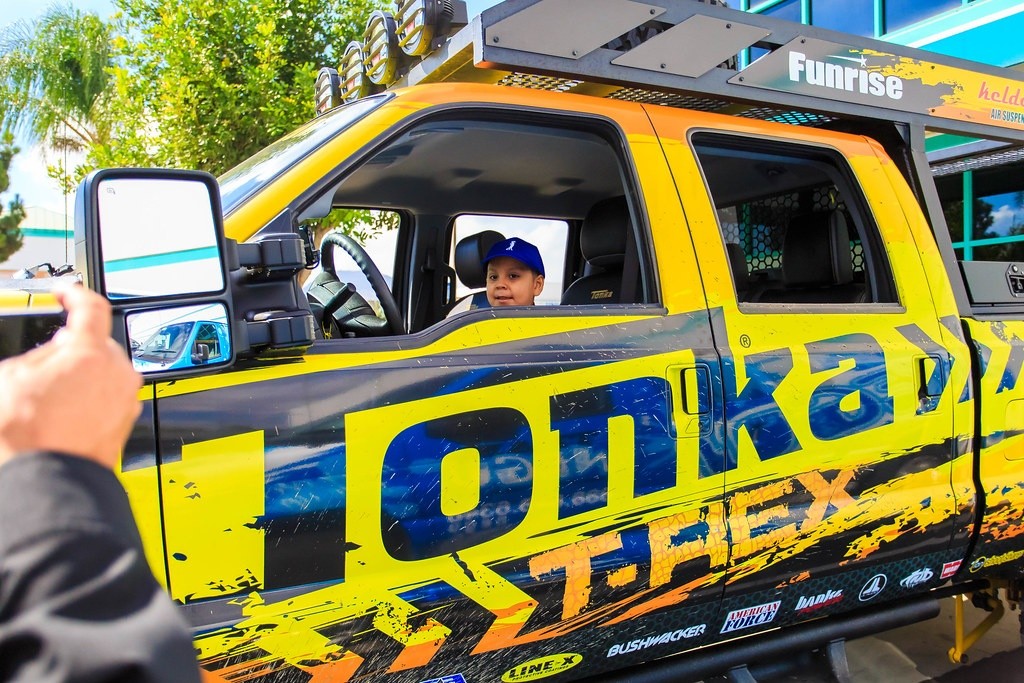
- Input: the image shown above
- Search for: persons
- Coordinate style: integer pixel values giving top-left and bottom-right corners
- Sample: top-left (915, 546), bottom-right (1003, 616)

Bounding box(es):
top-left (481, 236), bottom-right (547, 307)
top-left (0, 276), bottom-right (203, 683)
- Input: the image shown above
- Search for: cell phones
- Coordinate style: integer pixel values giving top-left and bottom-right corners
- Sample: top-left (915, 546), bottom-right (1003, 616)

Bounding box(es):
top-left (0, 308), bottom-right (66, 363)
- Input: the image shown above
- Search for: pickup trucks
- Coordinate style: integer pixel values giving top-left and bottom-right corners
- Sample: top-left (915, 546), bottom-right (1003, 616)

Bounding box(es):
top-left (0, 0), bottom-right (1024, 683)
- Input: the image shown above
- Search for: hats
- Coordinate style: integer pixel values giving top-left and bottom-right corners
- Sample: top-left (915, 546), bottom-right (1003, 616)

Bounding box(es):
top-left (483, 237), bottom-right (545, 277)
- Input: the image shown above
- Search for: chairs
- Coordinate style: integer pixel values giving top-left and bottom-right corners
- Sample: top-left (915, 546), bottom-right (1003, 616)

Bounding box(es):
top-left (169, 334), bottom-right (196, 358)
top-left (430, 229), bottom-right (507, 327)
top-left (726, 240), bottom-right (776, 302)
top-left (780, 207), bottom-right (868, 304)
top-left (557, 206), bottom-right (646, 307)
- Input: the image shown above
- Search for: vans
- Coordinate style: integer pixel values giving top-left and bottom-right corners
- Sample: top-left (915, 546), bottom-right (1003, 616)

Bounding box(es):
top-left (130, 321), bottom-right (231, 372)
